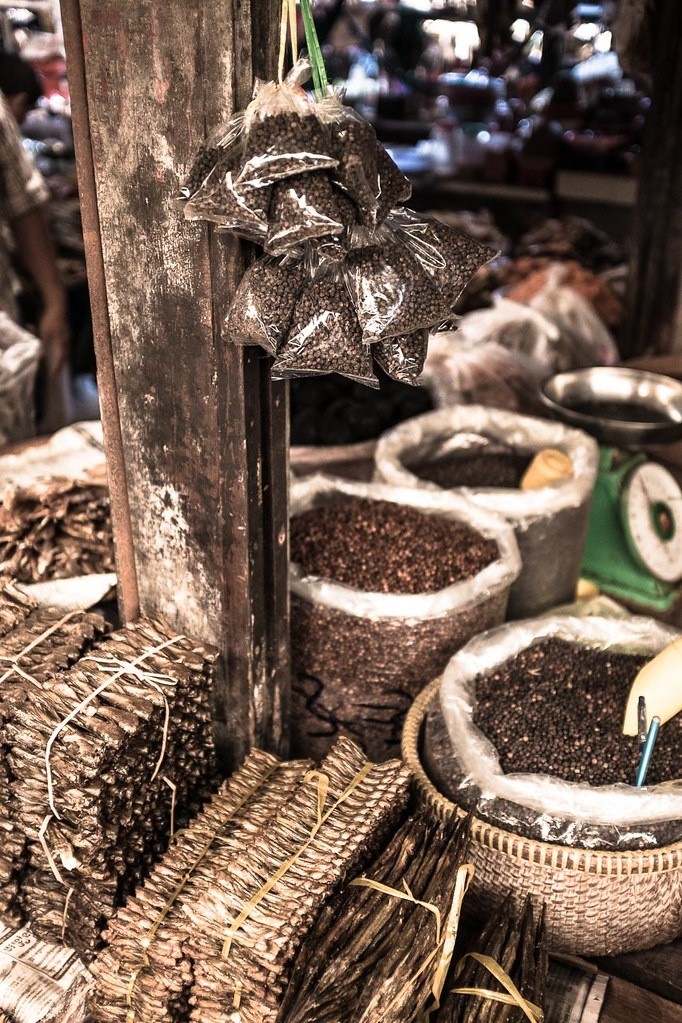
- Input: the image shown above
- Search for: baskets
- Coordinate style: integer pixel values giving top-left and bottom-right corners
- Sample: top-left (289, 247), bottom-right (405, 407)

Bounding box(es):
top-left (401, 675), bottom-right (682, 958)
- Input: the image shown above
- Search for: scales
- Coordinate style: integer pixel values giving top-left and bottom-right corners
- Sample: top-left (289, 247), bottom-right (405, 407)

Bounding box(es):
top-left (538, 365), bottom-right (682, 612)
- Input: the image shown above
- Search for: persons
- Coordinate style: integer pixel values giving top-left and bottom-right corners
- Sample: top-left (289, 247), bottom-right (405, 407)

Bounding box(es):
top-left (1, 54), bottom-right (70, 374)
